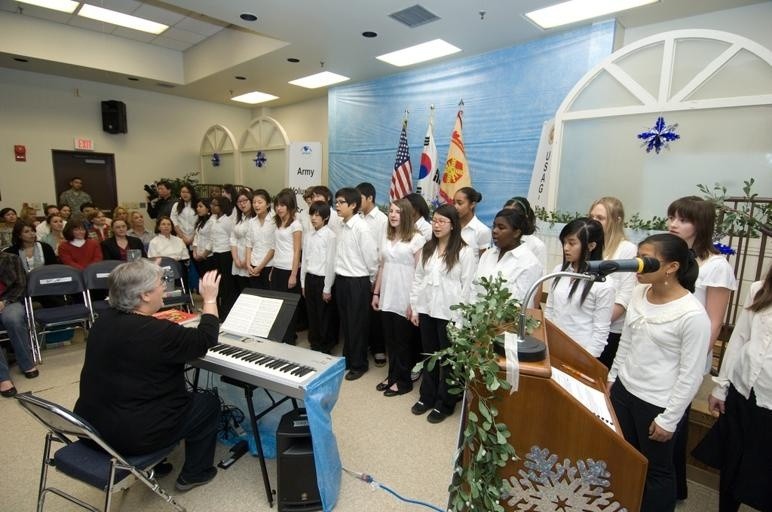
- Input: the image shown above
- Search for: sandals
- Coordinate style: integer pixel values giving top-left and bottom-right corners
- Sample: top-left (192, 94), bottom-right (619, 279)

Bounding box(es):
top-left (24, 368), bottom-right (39, 378)
top-left (383, 388), bottom-right (401, 397)
top-left (1, 379), bottom-right (18, 398)
top-left (375, 380), bottom-right (387, 391)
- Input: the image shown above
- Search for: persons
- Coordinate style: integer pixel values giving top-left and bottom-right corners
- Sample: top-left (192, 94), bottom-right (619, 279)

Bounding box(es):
top-left (608, 233), bottom-right (712, 512)
top-left (665, 195), bottom-right (737, 501)
top-left (705, 265), bottom-right (771, 511)
top-left (72, 258), bottom-right (223, 493)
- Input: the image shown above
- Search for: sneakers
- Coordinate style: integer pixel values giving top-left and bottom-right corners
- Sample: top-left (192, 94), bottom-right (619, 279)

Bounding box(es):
top-left (374, 351), bottom-right (387, 367)
top-left (428, 408), bottom-right (449, 423)
top-left (411, 402), bottom-right (430, 416)
top-left (411, 369), bottom-right (422, 381)
top-left (174, 465), bottom-right (218, 492)
top-left (344, 364), bottom-right (368, 381)
top-left (146, 462), bottom-right (173, 478)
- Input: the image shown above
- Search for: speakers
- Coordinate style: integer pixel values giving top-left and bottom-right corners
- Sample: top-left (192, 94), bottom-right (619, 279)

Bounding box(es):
top-left (100, 100), bottom-right (127, 134)
top-left (277, 407), bottom-right (324, 511)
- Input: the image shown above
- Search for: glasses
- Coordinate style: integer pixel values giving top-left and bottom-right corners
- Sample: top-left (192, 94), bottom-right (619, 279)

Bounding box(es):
top-left (429, 217), bottom-right (453, 226)
top-left (334, 199), bottom-right (349, 204)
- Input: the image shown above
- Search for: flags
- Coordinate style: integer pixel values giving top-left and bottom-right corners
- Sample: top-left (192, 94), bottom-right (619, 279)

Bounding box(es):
top-left (437, 111), bottom-right (472, 207)
top-left (414, 125), bottom-right (442, 211)
top-left (387, 123), bottom-right (414, 203)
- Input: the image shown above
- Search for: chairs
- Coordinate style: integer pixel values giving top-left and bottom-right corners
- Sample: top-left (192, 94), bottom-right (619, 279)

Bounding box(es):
top-left (12, 390), bottom-right (187, 511)
top-left (0, 241), bottom-right (197, 371)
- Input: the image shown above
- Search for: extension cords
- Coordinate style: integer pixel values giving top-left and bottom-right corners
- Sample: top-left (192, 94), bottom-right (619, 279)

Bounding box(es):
top-left (229, 419), bottom-right (247, 436)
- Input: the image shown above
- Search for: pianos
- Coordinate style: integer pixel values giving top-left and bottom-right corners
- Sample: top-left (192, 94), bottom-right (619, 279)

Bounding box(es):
top-left (183, 321), bottom-right (347, 401)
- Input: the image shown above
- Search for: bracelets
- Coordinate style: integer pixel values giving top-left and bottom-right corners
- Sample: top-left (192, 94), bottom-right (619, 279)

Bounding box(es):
top-left (204, 299), bottom-right (217, 305)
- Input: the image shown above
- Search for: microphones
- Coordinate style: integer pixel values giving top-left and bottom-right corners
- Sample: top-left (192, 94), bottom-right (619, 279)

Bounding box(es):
top-left (583, 257), bottom-right (660, 274)
